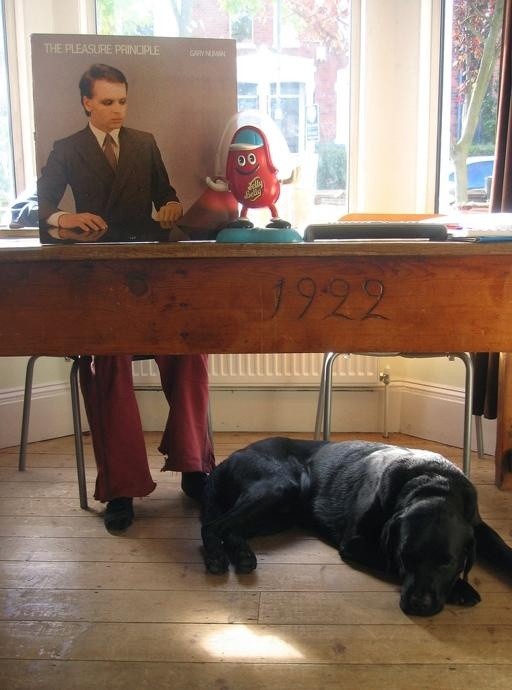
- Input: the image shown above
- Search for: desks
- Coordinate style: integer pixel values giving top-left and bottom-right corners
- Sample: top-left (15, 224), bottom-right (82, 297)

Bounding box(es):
top-left (1, 238), bottom-right (512, 491)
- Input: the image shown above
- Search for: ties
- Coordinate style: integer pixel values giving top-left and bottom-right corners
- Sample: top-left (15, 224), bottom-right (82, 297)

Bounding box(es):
top-left (104, 135), bottom-right (117, 172)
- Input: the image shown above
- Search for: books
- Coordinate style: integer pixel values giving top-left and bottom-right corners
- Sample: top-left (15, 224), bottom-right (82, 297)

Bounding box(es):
top-left (449, 236), bottom-right (512, 244)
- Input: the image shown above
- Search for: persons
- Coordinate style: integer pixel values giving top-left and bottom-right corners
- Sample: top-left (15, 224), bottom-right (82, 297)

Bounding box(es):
top-left (37, 61), bottom-right (186, 235)
top-left (9, 190), bottom-right (216, 538)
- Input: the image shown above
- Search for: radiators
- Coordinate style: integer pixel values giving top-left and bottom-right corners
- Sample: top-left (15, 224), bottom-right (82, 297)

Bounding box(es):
top-left (133, 353), bottom-right (389, 439)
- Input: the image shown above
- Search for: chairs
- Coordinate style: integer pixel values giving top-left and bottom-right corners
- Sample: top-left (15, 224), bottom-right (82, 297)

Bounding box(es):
top-left (314, 214), bottom-right (484, 478)
top-left (19, 355), bottom-right (212, 510)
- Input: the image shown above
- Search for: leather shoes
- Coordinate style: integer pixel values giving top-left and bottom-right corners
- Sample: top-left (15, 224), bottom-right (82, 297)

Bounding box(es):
top-left (103, 496), bottom-right (134, 533)
top-left (181, 471), bottom-right (208, 499)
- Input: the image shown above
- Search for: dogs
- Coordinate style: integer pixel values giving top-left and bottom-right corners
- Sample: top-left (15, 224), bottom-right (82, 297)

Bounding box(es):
top-left (199, 434), bottom-right (512, 617)
